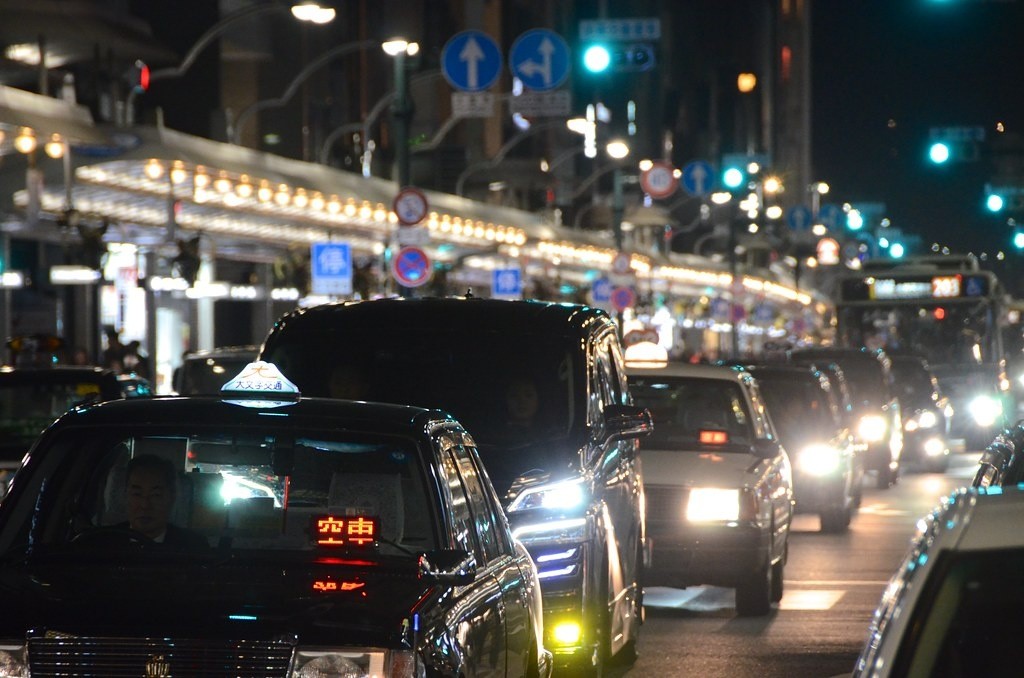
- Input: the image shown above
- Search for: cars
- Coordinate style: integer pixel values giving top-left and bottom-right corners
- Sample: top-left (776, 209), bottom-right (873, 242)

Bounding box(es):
top-left (0, 358), bottom-right (548, 677)
top-left (691, 340), bottom-right (1024, 678)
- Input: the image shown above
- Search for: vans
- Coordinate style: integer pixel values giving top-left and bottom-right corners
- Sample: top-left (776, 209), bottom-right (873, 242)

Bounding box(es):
top-left (1, 363), bottom-right (124, 459)
top-left (257, 292), bottom-right (656, 677)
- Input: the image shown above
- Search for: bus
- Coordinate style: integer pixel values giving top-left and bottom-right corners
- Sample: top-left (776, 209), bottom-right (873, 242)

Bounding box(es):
top-left (830, 255), bottom-right (1012, 445)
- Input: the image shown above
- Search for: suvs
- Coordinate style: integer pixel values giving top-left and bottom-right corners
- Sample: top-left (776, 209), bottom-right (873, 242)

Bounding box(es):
top-left (629, 360), bottom-right (796, 615)
